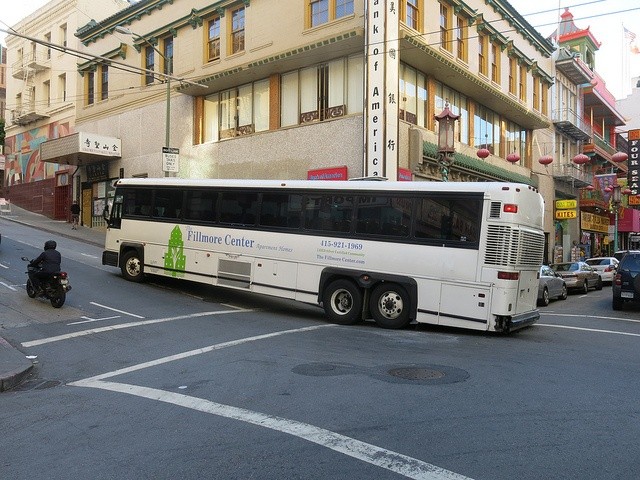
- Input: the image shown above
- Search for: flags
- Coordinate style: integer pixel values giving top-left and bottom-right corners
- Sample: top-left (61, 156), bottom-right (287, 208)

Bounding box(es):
top-left (623, 26), bottom-right (636, 46)
top-left (630, 44), bottom-right (640, 55)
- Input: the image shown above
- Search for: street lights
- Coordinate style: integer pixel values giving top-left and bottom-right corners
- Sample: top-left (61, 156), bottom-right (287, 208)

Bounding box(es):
top-left (608, 183), bottom-right (626, 252)
top-left (114, 25), bottom-right (172, 178)
top-left (433, 97), bottom-right (462, 182)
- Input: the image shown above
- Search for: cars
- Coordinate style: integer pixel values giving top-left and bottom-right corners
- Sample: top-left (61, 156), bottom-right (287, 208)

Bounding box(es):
top-left (548, 261), bottom-right (603, 294)
top-left (583, 256), bottom-right (620, 286)
top-left (537, 264), bottom-right (568, 306)
top-left (613, 250), bottom-right (640, 261)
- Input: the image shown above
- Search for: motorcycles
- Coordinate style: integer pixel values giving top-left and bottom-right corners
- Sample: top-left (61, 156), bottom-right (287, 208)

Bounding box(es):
top-left (21, 256), bottom-right (72, 309)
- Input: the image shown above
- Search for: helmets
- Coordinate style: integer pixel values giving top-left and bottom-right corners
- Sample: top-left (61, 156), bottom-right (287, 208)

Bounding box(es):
top-left (44, 240), bottom-right (57, 251)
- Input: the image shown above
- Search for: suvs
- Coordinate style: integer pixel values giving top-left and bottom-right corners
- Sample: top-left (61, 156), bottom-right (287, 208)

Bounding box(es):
top-left (612, 249), bottom-right (640, 310)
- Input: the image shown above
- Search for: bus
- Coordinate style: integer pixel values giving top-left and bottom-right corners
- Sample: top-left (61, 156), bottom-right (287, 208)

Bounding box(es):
top-left (102, 178), bottom-right (546, 334)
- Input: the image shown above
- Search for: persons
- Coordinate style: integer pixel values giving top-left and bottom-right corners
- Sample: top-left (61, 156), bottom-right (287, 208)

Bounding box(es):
top-left (32, 239), bottom-right (62, 297)
top-left (70, 199), bottom-right (80, 230)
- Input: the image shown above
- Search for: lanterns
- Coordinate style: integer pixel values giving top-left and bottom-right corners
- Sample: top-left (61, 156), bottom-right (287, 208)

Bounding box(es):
top-left (604, 188), bottom-right (611, 192)
top-left (586, 185), bottom-right (594, 191)
top-left (624, 188), bottom-right (632, 194)
top-left (538, 155), bottom-right (553, 174)
top-left (611, 151), bottom-right (629, 164)
top-left (476, 148), bottom-right (490, 159)
top-left (573, 153), bottom-right (589, 167)
top-left (506, 153), bottom-right (521, 166)
